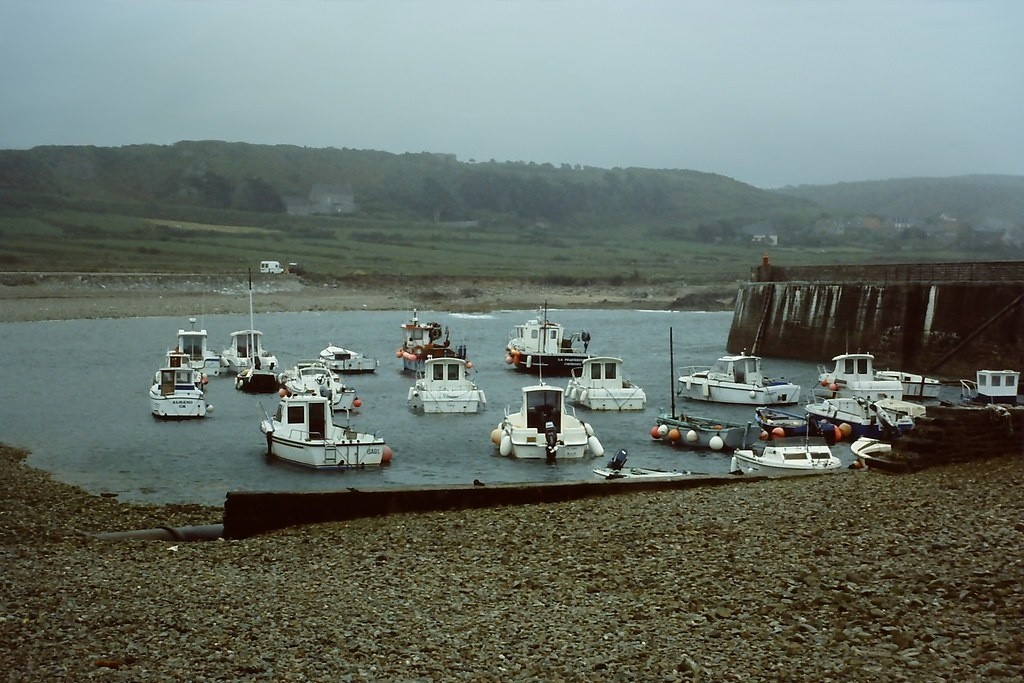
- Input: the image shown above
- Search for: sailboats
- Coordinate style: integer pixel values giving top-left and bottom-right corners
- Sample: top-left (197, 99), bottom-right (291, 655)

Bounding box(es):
top-left (650, 326), bottom-right (763, 450)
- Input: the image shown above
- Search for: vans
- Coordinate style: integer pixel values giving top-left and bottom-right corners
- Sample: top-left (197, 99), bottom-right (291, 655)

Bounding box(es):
top-left (260, 261), bottom-right (284, 274)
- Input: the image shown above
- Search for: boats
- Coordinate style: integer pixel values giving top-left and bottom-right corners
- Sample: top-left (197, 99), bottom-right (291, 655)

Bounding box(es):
top-left (803, 353), bottom-right (1024, 469)
top-left (148, 350), bottom-right (214, 418)
top-left (675, 347), bottom-right (802, 406)
top-left (260, 393), bottom-right (385, 470)
top-left (592, 449), bottom-right (710, 479)
top-left (506, 309), bottom-right (595, 375)
top-left (222, 330), bottom-right (279, 373)
top-left (278, 362), bottom-right (341, 384)
top-left (729, 446), bottom-right (843, 474)
top-left (755, 406), bottom-right (841, 443)
top-left (489, 383), bottom-right (605, 459)
top-left (281, 367), bottom-right (357, 411)
top-left (563, 356), bottom-right (647, 411)
top-left (397, 309), bottom-right (467, 374)
top-left (320, 342), bottom-right (378, 373)
top-left (407, 355), bottom-right (488, 414)
top-left (165, 317), bottom-right (229, 376)
top-left (234, 367), bottom-right (278, 391)
